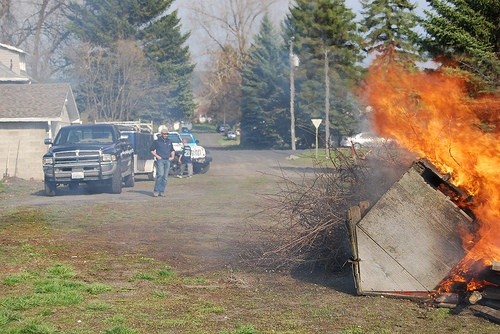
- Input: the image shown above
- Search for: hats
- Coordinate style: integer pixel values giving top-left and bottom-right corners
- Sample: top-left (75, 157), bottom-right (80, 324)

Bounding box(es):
top-left (161, 129), bottom-right (169, 133)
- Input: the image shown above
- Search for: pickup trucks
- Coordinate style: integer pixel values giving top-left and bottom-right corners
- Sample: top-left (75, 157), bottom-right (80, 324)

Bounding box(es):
top-left (42, 123), bottom-right (136, 196)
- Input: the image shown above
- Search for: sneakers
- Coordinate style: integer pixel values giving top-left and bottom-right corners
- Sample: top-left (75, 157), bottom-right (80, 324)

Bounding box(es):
top-left (186, 176), bottom-right (191, 179)
top-left (176, 174), bottom-right (182, 179)
top-left (159, 192), bottom-right (164, 197)
top-left (153, 190), bottom-right (159, 197)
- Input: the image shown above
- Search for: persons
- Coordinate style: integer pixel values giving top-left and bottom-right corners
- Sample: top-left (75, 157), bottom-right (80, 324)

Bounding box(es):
top-left (150, 129), bottom-right (175, 197)
top-left (177, 139), bottom-right (192, 178)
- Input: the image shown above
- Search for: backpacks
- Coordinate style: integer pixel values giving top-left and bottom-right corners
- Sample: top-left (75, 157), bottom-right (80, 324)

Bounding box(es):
top-left (181, 146), bottom-right (191, 163)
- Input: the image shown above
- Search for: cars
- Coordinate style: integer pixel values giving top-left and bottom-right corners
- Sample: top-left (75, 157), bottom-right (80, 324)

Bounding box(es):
top-left (227, 131), bottom-right (237, 140)
top-left (341, 132), bottom-right (398, 151)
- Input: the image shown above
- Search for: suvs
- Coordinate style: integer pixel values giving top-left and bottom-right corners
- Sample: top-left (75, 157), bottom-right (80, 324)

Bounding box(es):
top-left (180, 132), bottom-right (211, 174)
top-left (98, 121), bottom-right (157, 178)
top-left (153, 132), bottom-right (189, 172)
